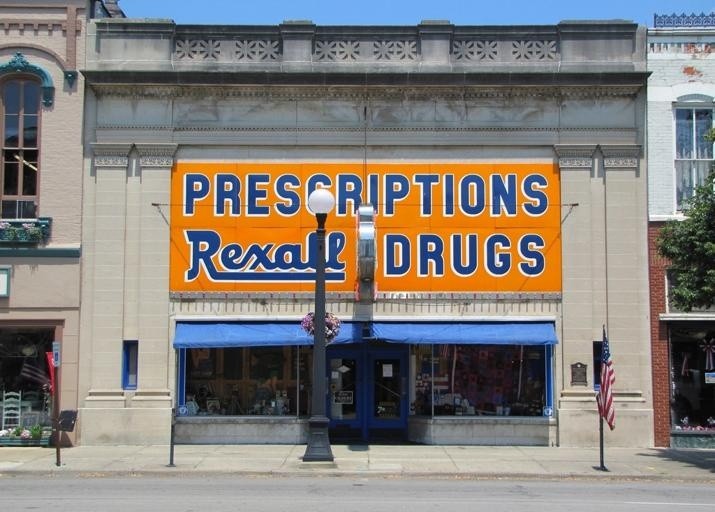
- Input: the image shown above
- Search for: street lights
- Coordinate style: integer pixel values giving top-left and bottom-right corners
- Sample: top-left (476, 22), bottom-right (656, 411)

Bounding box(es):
top-left (297, 187), bottom-right (339, 464)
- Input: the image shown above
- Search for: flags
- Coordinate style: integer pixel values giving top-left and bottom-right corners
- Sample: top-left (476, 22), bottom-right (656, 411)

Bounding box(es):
top-left (595, 324), bottom-right (617, 432)
